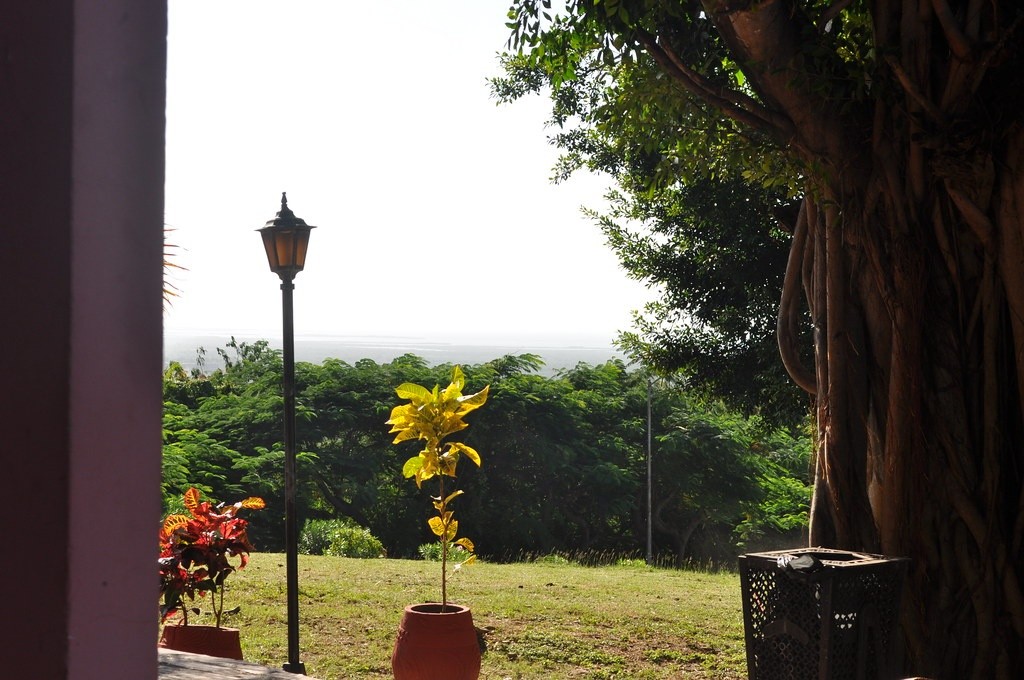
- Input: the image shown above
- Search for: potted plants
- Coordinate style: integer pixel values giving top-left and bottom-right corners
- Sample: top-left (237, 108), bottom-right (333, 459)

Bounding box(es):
top-left (383, 365), bottom-right (489, 680)
top-left (158, 487), bottom-right (265, 659)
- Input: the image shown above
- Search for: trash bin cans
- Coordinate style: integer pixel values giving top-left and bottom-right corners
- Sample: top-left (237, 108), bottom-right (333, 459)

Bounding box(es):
top-left (738, 546), bottom-right (911, 680)
